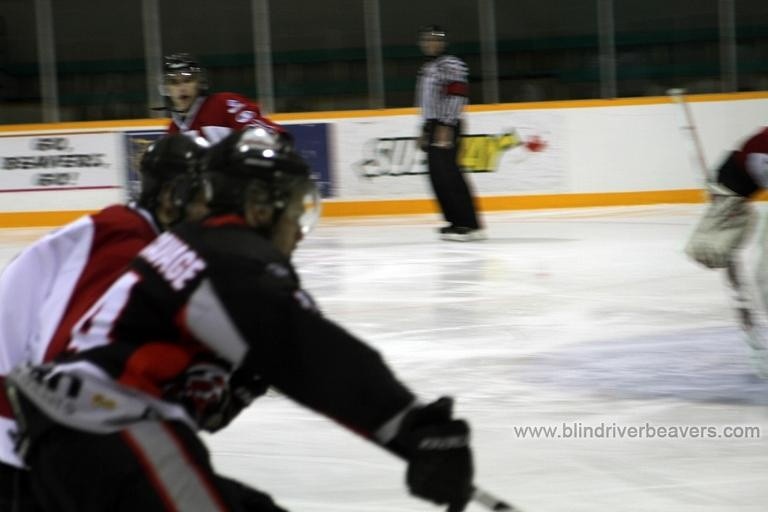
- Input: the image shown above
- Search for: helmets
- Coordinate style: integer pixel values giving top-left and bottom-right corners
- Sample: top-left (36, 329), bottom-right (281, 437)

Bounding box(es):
top-left (417, 23), bottom-right (449, 40)
top-left (156, 51), bottom-right (208, 95)
top-left (144, 128), bottom-right (219, 221)
top-left (202, 125), bottom-right (325, 240)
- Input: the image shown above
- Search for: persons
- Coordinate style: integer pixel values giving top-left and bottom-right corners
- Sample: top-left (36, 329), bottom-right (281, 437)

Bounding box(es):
top-left (413, 23), bottom-right (483, 236)
top-left (159, 49), bottom-right (296, 153)
top-left (690, 127), bottom-right (766, 321)
top-left (8, 128), bottom-right (476, 512)
top-left (0, 132), bottom-right (217, 512)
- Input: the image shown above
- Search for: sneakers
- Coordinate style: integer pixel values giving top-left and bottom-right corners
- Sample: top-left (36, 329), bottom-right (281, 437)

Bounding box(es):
top-left (438, 222), bottom-right (477, 234)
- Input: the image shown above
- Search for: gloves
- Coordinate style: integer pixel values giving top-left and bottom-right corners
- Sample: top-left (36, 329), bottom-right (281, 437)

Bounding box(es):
top-left (400, 398), bottom-right (475, 511)
top-left (432, 125), bottom-right (453, 149)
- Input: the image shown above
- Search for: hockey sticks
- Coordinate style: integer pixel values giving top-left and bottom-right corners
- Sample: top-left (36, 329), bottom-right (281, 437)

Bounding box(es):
top-left (669, 89), bottom-right (768, 350)
